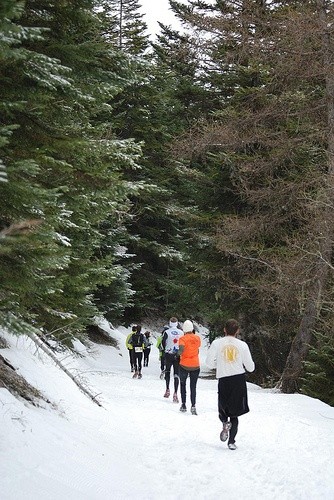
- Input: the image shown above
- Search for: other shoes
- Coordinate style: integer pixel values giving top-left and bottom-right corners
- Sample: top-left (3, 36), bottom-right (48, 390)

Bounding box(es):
top-left (173, 393), bottom-right (179, 403)
top-left (144, 364), bottom-right (145, 367)
top-left (131, 368), bottom-right (133, 372)
top-left (146, 364), bottom-right (148, 367)
top-left (160, 371), bottom-right (164, 379)
top-left (138, 374), bottom-right (142, 379)
top-left (164, 390), bottom-right (170, 398)
top-left (133, 372), bottom-right (138, 378)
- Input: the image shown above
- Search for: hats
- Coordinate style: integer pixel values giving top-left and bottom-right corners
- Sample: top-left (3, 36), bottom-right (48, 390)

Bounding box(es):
top-left (183, 320), bottom-right (194, 332)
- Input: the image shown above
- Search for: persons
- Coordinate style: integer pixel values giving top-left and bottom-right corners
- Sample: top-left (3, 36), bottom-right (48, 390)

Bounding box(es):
top-left (205, 319), bottom-right (255, 450)
top-left (125, 317), bottom-right (201, 415)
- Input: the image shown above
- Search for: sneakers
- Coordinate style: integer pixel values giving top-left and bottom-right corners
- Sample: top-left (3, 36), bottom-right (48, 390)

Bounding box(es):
top-left (220, 423), bottom-right (232, 441)
top-left (228, 443), bottom-right (236, 449)
top-left (190, 407), bottom-right (196, 415)
top-left (179, 407), bottom-right (186, 412)
